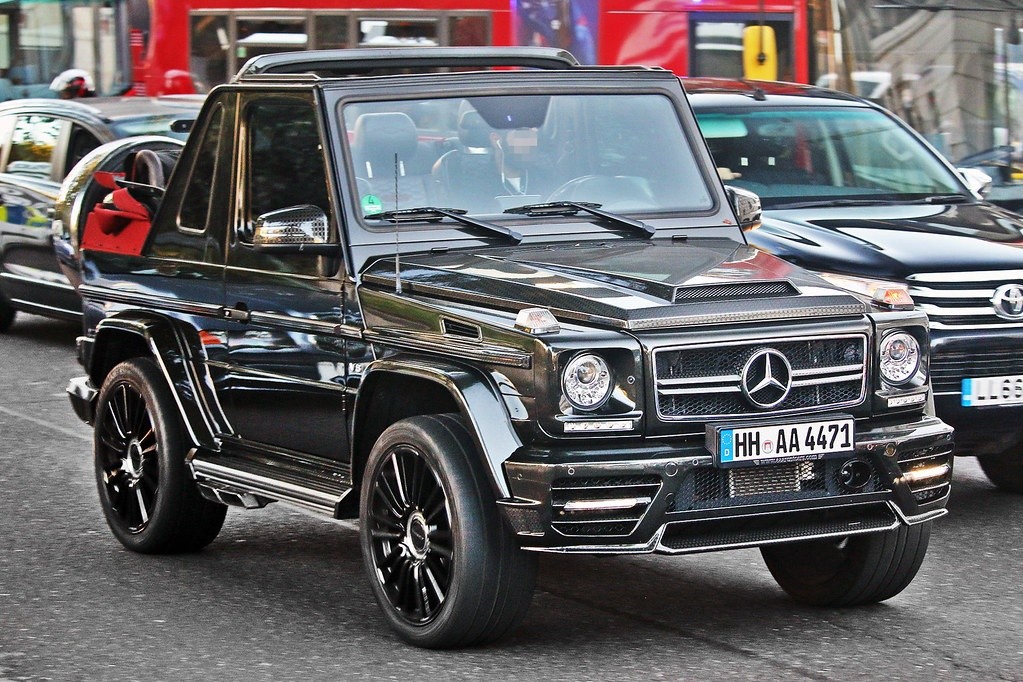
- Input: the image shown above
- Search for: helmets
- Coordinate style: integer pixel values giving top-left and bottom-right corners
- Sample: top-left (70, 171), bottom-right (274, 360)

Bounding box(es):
top-left (58, 69), bottom-right (95, 98)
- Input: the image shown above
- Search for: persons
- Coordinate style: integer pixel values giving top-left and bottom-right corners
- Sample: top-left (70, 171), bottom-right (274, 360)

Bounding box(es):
top-left (480, 124), bottom-right (571, 213)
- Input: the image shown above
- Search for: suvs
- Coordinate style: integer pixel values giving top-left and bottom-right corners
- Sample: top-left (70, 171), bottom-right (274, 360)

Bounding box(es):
top-left (63, 46), bottom-right (955, 653)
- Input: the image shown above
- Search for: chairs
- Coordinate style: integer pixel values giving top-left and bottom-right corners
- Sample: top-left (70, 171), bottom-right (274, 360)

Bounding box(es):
top-left (352, 113), bottom-right (428, 210)
top-left (433, 111), bottom-right (522, 215)
top-left (733, 133), bottom-right (787, 185)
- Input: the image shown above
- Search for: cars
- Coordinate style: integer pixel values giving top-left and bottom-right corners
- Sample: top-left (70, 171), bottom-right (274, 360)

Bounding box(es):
top-left (0, 94), bottom-right (232, 331)
top-left (642, 78), bottom-right (1023, 494)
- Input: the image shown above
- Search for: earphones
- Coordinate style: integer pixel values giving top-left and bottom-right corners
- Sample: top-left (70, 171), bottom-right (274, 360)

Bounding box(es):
top-left (496, 140), bottom-right (502, 151)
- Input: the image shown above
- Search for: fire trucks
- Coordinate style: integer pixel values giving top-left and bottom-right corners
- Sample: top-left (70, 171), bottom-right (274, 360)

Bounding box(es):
top-left (104, 1), bottom-right (814, 177)
